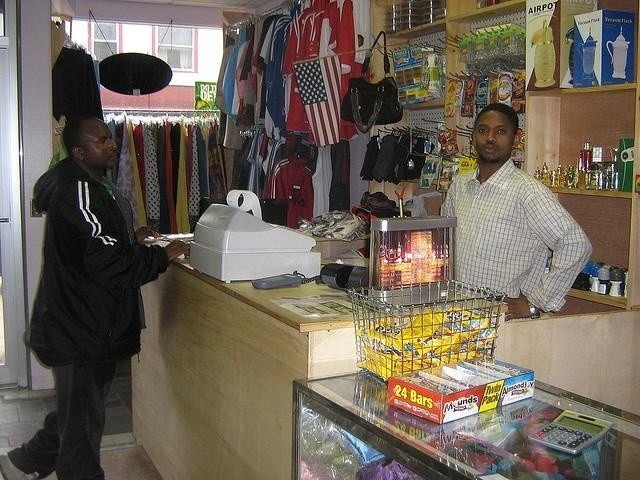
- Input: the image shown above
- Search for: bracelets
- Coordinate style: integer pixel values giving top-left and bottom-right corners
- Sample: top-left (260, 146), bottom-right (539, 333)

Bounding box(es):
top-left (527, 300), bottom-right (539, 320)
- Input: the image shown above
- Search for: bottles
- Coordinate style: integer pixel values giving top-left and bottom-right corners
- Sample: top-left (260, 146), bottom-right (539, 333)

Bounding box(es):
top-left (578, 259), bottom-right (627, 297)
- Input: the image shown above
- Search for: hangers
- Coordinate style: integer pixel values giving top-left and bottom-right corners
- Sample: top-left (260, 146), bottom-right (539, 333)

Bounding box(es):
top-left (62, 33), bottom-right (99, 59)
top-left (227, 0), bottom-right (305, 31)
top-left (102, 109), bottom-right (220, 129)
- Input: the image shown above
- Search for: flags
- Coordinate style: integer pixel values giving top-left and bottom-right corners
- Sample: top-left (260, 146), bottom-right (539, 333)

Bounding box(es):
top-left (293, 56), bottom-right (359, 147)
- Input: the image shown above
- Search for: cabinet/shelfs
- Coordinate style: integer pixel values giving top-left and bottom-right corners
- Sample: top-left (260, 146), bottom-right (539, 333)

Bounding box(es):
top-left (290, 366), bottom-right (638, 478)
top-left (369, 1), bottom-right (639, 312)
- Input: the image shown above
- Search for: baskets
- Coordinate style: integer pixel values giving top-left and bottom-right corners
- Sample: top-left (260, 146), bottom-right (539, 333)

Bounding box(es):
top-left (348, 278), bottom-right (506, 384)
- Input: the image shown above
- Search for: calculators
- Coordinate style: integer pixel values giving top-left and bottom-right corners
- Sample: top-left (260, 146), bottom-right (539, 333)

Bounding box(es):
top-left (528, 410), bottom-right (614, 455)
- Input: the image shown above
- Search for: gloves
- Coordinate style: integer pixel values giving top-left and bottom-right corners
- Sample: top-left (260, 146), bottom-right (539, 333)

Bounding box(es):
top-left (360, 133), bottom-right (435, 185)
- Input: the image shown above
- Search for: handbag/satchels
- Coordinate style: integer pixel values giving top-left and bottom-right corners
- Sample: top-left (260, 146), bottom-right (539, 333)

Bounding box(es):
top-left (340, 76), bottom-right (402, 125)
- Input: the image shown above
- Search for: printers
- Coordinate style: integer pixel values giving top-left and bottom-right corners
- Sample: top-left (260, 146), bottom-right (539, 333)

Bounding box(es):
top-left (189, 203), bottom-right (322, 284)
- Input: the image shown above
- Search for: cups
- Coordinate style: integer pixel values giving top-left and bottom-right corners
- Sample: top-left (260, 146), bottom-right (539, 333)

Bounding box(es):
top-left (578, 26), bottom-right (629, 79)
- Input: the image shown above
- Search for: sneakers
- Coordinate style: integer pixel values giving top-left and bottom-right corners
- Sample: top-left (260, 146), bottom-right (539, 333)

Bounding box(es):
top-left (360, 191), bottom-right (396, 209)
top-left (0, 455), bottom-right (39, 480)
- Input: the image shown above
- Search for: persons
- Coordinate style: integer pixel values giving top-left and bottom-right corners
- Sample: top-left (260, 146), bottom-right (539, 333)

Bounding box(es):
top-left (440, 103), bottom-right (592, 323)
top-left (5, 113), bottom-right (189, 480)
